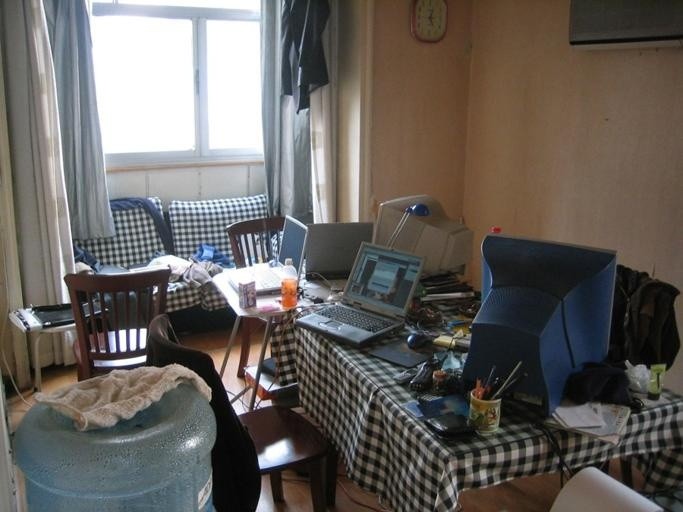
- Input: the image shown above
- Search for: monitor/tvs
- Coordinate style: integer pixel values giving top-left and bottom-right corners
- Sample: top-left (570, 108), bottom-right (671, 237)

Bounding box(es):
top-left (373, 194), bottom-right (477, 278)
top-left (461, 230), bottom-right (619, 420)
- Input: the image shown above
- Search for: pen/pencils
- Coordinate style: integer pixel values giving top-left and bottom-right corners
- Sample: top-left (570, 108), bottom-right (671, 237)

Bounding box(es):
top-left (475, 360), bottom-right (529, 400)
top-left (447, 320), bottom-right (470, 327)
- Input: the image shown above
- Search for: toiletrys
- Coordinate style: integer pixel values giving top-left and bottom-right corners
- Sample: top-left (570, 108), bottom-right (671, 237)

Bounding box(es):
top-left (647, 363), bottom-right (667, 400)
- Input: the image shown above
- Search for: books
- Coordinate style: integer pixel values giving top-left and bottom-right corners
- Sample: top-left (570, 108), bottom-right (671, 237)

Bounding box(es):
top-left (542, 405), bottom-right (633, 444)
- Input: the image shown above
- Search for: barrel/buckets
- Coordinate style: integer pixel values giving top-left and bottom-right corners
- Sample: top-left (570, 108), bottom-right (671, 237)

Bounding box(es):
top-left (11, 374), bottom-right (217, 510)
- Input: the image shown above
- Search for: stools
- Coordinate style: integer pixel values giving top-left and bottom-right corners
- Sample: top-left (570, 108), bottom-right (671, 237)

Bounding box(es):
top-left (32, 324), bottom-right (80, 393)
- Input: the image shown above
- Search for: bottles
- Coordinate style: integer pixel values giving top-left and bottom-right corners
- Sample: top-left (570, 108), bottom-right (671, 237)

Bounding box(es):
top-left (481, 225), bottom-right (503, 300)
top-left (280, 259), bottom-right (297, 308)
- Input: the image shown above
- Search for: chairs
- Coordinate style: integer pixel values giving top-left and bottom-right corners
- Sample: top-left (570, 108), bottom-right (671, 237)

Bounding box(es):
top-left (227, 217), bottom-right (285, 381)
top-left (75, 196), bottom-right (200, 328)
top-left (169, 195), bottom-right (269, 312)
top-left (146, 335), bottom-right (337, 512)
top-left (64, 267), bottom-right (174, 384)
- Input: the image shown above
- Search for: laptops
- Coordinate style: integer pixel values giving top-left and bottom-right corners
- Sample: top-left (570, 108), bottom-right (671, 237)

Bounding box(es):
top-left (304, 219), bottom-right (373, 279)
top-left (225, 216), bottom-right (308, 299)
top-left (295, 239), bottom-right (426, 350)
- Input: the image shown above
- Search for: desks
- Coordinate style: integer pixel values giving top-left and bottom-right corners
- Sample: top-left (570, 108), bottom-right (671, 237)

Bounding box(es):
top-left (271, 295), bottom-right (683, 512)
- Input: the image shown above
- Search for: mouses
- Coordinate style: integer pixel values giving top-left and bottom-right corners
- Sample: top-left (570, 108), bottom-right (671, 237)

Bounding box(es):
top-left (406, 329), bottom-right (433, 350)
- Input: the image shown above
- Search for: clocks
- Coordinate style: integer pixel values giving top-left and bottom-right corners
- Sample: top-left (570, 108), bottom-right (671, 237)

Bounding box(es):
top-left (410, 1), bottom-right (449, 42)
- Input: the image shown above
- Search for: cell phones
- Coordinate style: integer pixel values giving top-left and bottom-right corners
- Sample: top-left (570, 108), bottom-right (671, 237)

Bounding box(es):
top-left (393, 366), bottom-right (417, 386)
top-left (412, 352), bottom-right (449, 390)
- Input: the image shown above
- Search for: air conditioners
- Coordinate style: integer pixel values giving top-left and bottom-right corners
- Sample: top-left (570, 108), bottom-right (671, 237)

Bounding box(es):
top-left (568, 1), bottom-right (683, 50)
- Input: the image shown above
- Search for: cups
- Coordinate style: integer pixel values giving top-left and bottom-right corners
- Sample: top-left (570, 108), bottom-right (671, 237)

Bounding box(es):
top-left (469, 389), bottom-right (500, 434)
